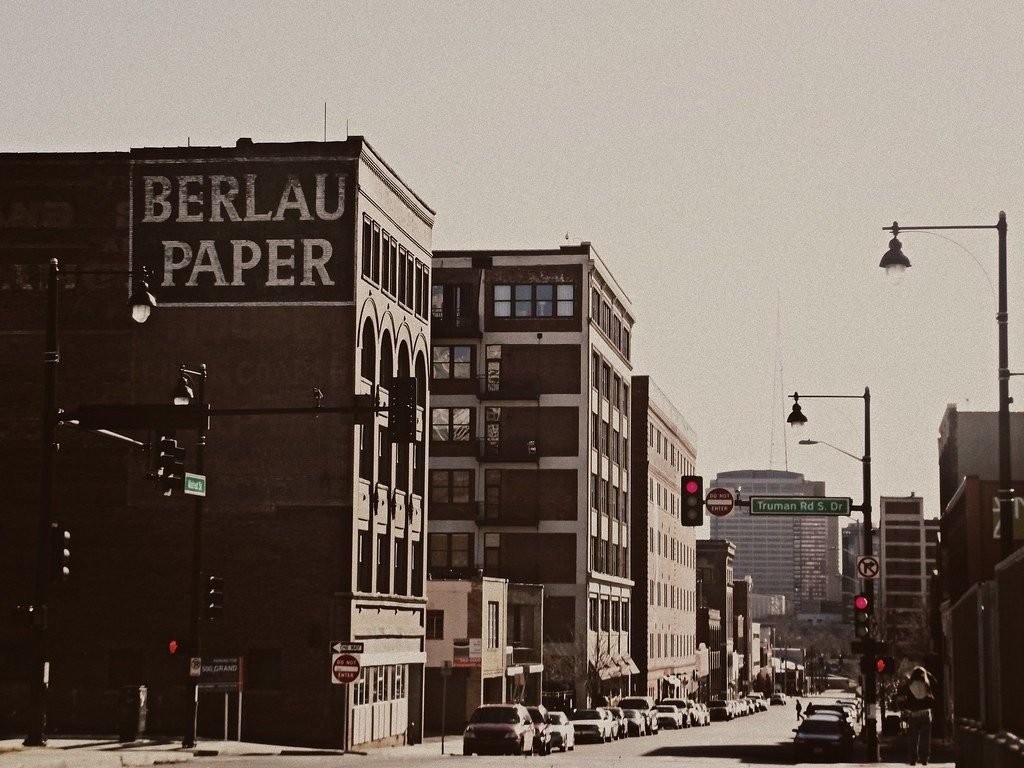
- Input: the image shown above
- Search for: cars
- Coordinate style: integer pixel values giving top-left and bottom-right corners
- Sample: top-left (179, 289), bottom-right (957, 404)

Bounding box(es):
top-left (791, 701), bottom-right (863, 762)
top-left (770, 693), bottom-right (786, 705)
top-left (707, 698), bottom-right (765, 722)
top-left (547, 707), bottom-right (645, 752)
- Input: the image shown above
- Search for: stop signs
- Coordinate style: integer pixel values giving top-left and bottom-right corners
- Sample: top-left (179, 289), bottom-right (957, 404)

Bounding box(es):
top-left (705, 488), bottom-right (733, 517)
top-left (331, 653), bottom-right (361, 684)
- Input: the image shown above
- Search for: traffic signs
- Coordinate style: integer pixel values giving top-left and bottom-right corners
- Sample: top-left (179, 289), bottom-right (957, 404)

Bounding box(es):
top-left (329, 641), bottom-right (364, 655)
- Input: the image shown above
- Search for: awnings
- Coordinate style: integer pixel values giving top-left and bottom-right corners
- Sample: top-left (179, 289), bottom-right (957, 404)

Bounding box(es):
top-left (589, 653), bottom-right (640, 681)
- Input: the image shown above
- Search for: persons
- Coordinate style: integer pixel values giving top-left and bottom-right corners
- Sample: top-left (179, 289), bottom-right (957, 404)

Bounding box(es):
top-left (796, 699), bottom-right (805, 721)
top-left (896, 666), bottom-right (938, 765)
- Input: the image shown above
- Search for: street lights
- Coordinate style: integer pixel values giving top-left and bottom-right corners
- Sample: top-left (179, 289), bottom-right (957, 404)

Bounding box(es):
top-left (172, 363), bottom-right (208, 747)
top-left (25, 255), bottom-right (159, 749)
top-left (787, 389), bottom-right (878, 749)
top-left (876, 211), bottom-right (1024, 739)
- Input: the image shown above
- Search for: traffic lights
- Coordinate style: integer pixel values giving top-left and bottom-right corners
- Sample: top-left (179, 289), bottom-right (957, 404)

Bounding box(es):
top-left (207, 575), bottom-right (225, 620)
top-left (876, 657), bottom-right (895, 674)
top-left (55, 526), bottom-right (72, 585)
top-left (164, 638), bottom-right (184, 664)
top-left (681, 475), bottom-right (703, 526)
top-left (853, 595), bottom-right (870, 637)
top-left (146, 432), bottom-right (186, 497)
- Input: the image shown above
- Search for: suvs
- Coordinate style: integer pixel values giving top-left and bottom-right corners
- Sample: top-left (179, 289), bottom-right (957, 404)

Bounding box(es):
top-left (463, 703), bottom-right (554, 756)
top-left (618, 696), bottom-right (710, 736)
